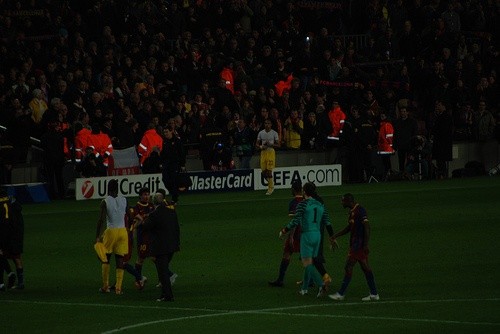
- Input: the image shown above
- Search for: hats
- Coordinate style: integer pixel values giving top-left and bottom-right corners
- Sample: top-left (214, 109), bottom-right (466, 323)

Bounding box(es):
top-left (343, 194), bottom-right (355, 209)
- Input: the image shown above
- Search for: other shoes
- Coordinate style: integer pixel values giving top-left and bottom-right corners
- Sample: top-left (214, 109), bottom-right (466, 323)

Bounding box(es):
top-left (267, 279), bottom-right (283, 286)
top-left (18, 283), bottom-right (24, 289)
top-left (0, 284), bottom-right (5, 291)
top-left (170, 273), bottom-right (178, 285)
top-left (134, 276), bottom-right (147, 289)
top-left (111, 283), bottom-right (116, 289)
top-left (265, 188), bottom-right (274, 196)
top-left (316, 279), bottom-right (329, 298)
top-left (99, 287), bottom-right (111, 293)
top-left (321, 274), bottom-right (331, 282)
top-left (299, 289), bottom-right (307, 294)
top-left (296, 280), bottom-right (303, 285)
top-left (116, 289), bottom-right (121, 295)
top-left (156, 297), bottom-right (175, 302)
top-left (7, 272), bottom-right (16, 288)
top-left (157, 282), bottom-right (163, 288)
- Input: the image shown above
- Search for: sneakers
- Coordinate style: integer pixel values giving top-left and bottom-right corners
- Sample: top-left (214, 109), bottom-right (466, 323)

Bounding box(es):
top-left (362, 294), bottom-right (379, 301)
top-left (328, 292), bottom-right (344, 301)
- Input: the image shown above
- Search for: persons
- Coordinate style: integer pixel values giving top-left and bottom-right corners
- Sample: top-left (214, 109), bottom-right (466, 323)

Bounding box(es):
top-left (256, 119), bottom-right (278, 197)
top-left (99, 179), bottom-right (128, 295)
top-left (269, 180), bottom-right (334, 299)
top-left (328, 193), bottom-right (380, 301)
top-left (0, 0), bottom-right (500, 190)
top-left (0, 191), bottom-right (25, 290)
top-left (144, 193), bottom-right (183, 303)
top-left (378, 112), bottom-right (395, 178)
top-left (29, 88), bottom-right (48, 122)
top-left (132, 187), bottom-right (177, 288)
